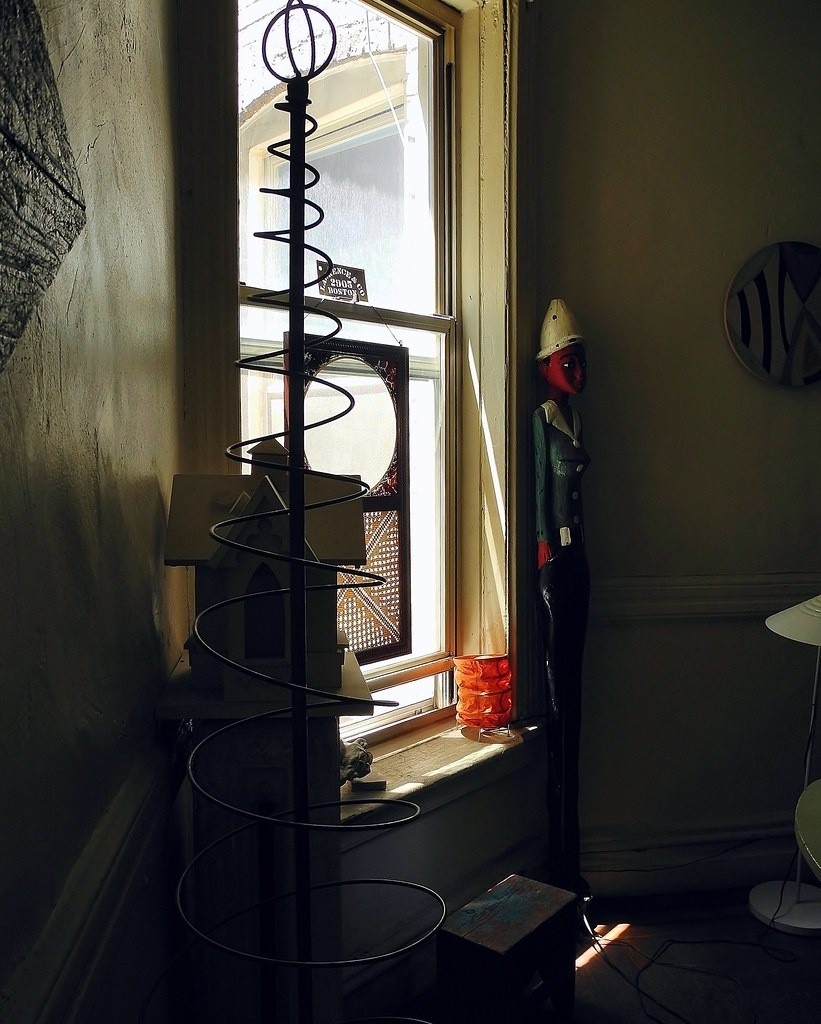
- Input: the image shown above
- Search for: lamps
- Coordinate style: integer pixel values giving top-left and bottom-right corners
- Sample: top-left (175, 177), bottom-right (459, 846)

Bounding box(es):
top-left (750, 593), bottom-right (820, 936)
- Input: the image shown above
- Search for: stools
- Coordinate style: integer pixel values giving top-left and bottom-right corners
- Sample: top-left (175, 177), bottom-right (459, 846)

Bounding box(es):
top-left (434, 870), bottom-right (576, 1023)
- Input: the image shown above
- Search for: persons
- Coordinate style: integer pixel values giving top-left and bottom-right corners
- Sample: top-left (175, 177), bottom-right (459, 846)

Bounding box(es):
top-left (530, 297), bottom-right (592, 898)
